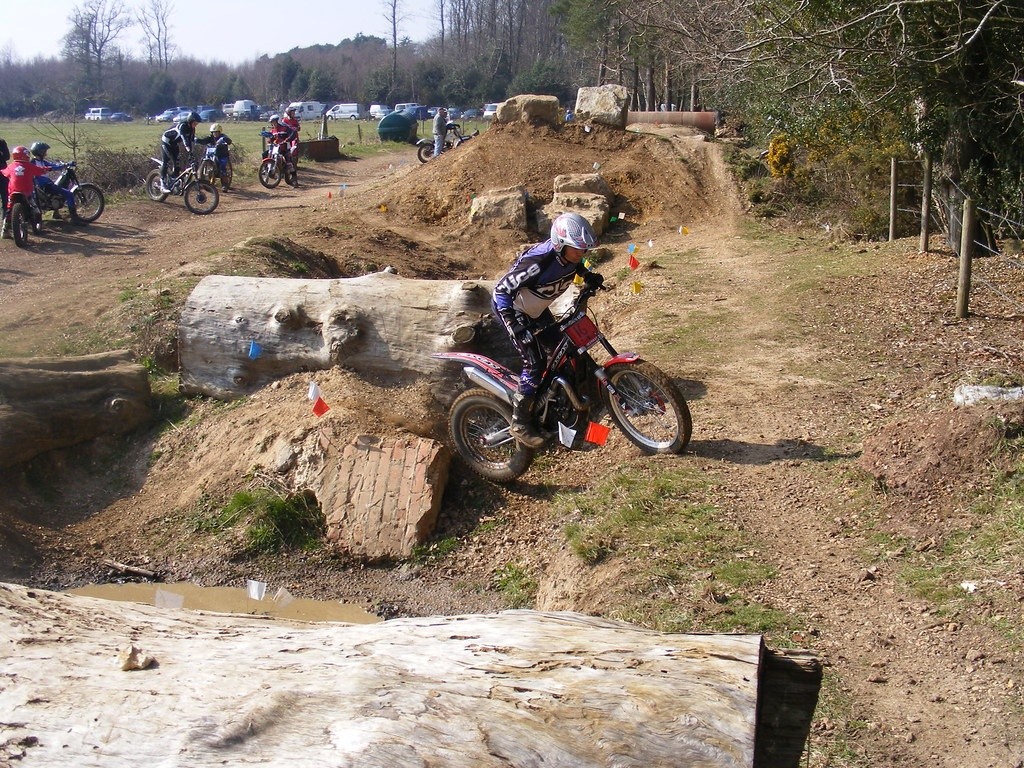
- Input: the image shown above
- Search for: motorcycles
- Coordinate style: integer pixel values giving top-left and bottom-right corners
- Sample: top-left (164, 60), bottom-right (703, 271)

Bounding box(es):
top-left (143, 151), bottom-right (220, 216)
top-left (415, 123), bottom-right (472, 163)
top-left (429, 281), bottom-right (693, 485)
top-left (5, 173), bottom-right (43, 249)
top-left (195, 143), bottom-right (234, 187)
top-left (258, 131), bottom-right (295, 189)
top-left (34, 162), bottom-right (106, 224)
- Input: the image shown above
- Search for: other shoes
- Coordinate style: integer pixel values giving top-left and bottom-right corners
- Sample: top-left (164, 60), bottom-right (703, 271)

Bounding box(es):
top-left (53, 212), bottom-right (64, 220)
top-left (290, 172), bottom-right (298, 182)
top-left (261, 169), bottom-right (268, 175)
top-left (70, 219), bottom-right (88, 227)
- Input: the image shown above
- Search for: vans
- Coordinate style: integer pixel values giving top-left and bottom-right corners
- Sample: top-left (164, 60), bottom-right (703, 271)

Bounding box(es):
top-left (283, 102), bottom-right (322, 122)
top-left (406, 106), bottom-right (426, 119)
top-left (85, 107), bottom-right (113, 121)
top-left (325, 103), bottom-right (361, 121)
top-left (395, 102), bottom-right (422, 112)
top-left (370, 105), bottom-right (393, 118)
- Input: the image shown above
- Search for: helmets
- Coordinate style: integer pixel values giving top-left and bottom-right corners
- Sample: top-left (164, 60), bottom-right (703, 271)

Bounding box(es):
top-left (12, 146), bottom-right (31, 163)
top-left (285, 106), bottom-right (296, 117)
top-left (208, 122), bottom-right (222, 133)
top-left (30, 142), bottom-right (50, 157)
top-left (268, 114), bottom-right (279, 123)
top-left (551, 212), bottom-right (599, 253)
top-left (186, 110), bottom-right (203, 124)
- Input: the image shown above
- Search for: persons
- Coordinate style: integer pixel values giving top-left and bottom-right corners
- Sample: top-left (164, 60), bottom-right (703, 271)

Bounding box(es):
top-left (0, 138), bottom-right (10, 219)
top-left (196, 123), bottom-right (232, 192)
top-left (433, 107), bottom-right (448, 157)
top-left (261, 106), bottom-right (301, 179)
top-left (159, 111), bottom-right (202, 194)
top-left (491, 212), bottom-right (604, 449)
top-left (566, 109), bottom-right (572, 121)
top-left (30, 142), bottom-right (87, 227)
top-left (0, 146), bottom-right (52, 239)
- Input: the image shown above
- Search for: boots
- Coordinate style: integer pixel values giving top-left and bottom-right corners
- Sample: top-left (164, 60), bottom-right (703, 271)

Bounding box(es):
top-left (159, 179), bottom-right (172, 194)
top-left (509, 391), bottom-right (544, 449)
top-left (0, 217), bottom-right (12, 239)
top-left (221, 172), bottom-right (228, 192)
top-left (35, 212), bottom-right (42, 235)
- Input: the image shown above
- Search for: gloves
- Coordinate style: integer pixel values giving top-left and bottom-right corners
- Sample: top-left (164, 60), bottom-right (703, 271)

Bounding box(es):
top-left (67, 160), bottom-right (77, 167)
top-left (506, 320), bottom-right (528, 340)
top-left (582, 271), bottom-right (608, 291)
top-left (44, 165), bottom-right (53, 173)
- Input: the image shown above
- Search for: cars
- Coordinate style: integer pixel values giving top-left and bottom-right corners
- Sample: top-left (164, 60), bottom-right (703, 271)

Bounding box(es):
top-left (156, 100), bottom-right (287, 122)
top-left (483, 103), bottom-right (498, 122)
top-left (427, 106), bottom-right (466, 120)
top-left (464, 108), bottom-right (482, 119)
top-left (110, 112), bottom-right (133, 122)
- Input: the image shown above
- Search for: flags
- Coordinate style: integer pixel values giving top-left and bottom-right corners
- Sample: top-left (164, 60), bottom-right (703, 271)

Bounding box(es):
top-left (633, 281), bottom-right (641, 294)
top-left (628, 243), bottom-right (640, 271)
top-left (610, 213), bottom-right (625, 222)
top-left (585, 125), bottom-right (590, 132)
top-left (574, 257), bottom-right (593, 285)
top-left (247, 580), bottom-right (295, 609)
top-left (558, 421), bottom-right (610, 447)
top-left (308, 381), bottom-right (330, 418)
top-left (249, 341), bottom-right (262, 361)
top-left (648, 240), bottom-right (653, 247)
top-left (679, 225), bottom-right (688, 236)
top-left (593, 162), bottom-right (600, 169)
